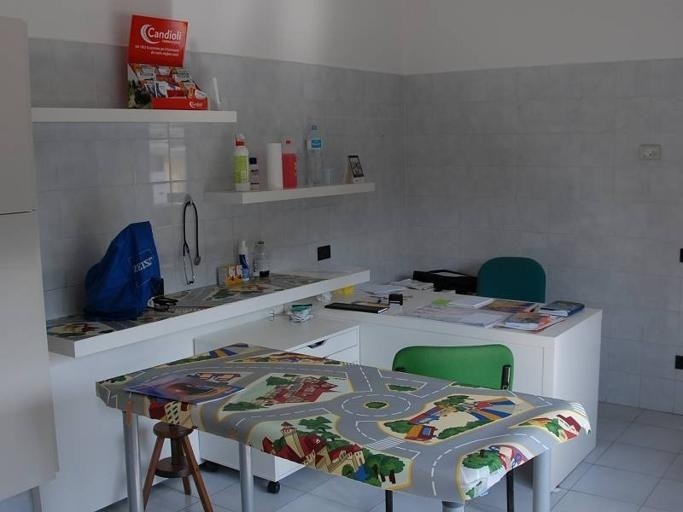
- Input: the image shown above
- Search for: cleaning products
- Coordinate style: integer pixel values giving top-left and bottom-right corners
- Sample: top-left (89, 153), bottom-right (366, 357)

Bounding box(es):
top-left (236, 239), bottom-right (251, 280)
top-left (232, 131), bottom-right (250, 191)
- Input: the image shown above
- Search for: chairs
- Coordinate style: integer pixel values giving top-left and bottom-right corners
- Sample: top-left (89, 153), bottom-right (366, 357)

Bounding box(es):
top-left (474, 256), bottom-right (546, 305)
top-left (383, 343), bottom-right (515, 512)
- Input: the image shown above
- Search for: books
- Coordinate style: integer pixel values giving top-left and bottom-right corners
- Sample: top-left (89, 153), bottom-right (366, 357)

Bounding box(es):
top-left (324, 279), bottom-right (585, 331)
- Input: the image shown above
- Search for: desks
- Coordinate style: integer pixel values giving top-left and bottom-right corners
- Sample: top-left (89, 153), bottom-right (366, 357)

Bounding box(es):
top-left (317, 283), bottom-right (604, 497)
top-left (94, 341), bottom-right (592, 512)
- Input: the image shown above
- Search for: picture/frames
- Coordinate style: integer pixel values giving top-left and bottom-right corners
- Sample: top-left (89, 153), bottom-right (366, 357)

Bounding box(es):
top-left (341, 154), bottom-right (366, 186)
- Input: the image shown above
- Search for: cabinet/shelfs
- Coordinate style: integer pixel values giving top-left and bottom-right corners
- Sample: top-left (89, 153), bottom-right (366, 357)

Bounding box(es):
top-left (192, 313), bottom-right (361, 495)
top-left (30, 106), bottom-right (377, 207)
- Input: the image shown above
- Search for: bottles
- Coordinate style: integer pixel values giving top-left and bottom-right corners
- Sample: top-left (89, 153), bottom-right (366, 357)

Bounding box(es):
top-left (230, 140), bottom-right (251, 193)
top-left (248, 157), bottom-right (262, 189)
top-left (253, 241), bottom-right (271, 276)
top-left (266, 141), bottom-right (282, 190)
top-left (238, 240), bottom-right (250, 279)
top-left (306, 125), bottom-right (324, 186)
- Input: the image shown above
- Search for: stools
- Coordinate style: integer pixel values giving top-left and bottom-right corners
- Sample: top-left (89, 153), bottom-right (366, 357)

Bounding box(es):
top-left (143, 422), bottom-right (214, 510)
